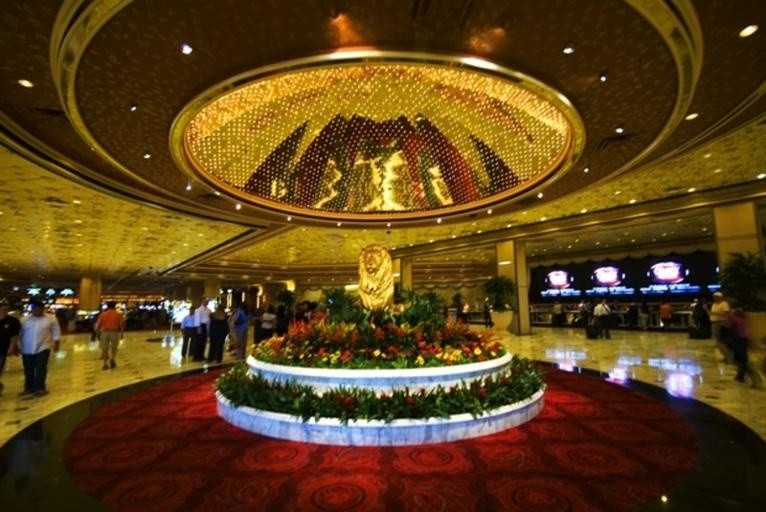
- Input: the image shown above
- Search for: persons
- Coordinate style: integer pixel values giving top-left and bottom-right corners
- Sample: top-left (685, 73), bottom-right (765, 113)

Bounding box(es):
top-left (660, 301), bottom-right (674, 331)
top-left (552, 302), bottom-right (563, 327)
top-left (456, 302), bottom-right (470, 324)
top-left (14, 296), bottom-right (62, 397)
top-left (593, 299), bottom-right (611, 339)
top-left (710, 293), bottom-right (766, 389)
top-left (182, 298), bottom-right (318, 364)
top-left (0, 296), bottom-right (23, 397)
top-left (92, 302), bottom-right (123, 369)
top-left (578, 300), bottom-right (588, 327)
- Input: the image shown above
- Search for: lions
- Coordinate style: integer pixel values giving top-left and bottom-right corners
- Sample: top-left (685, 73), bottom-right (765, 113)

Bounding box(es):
top-left (342, 243), bottom-right (412, 318)
